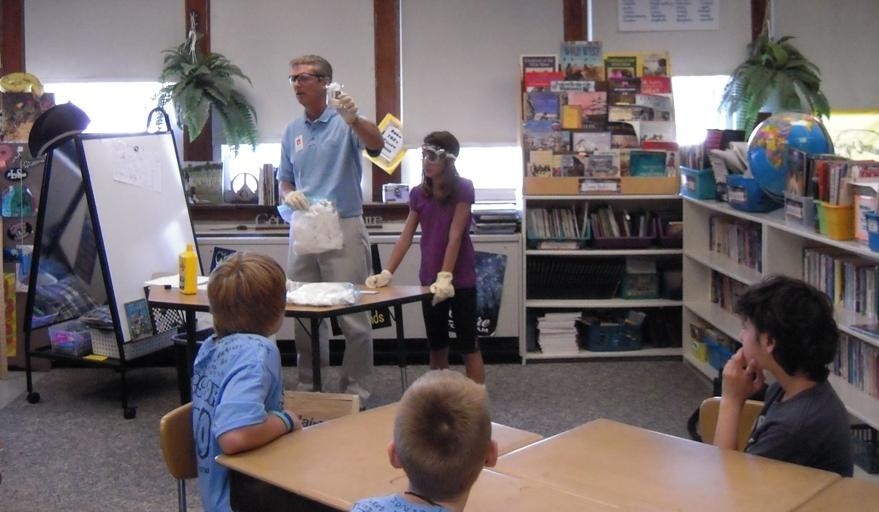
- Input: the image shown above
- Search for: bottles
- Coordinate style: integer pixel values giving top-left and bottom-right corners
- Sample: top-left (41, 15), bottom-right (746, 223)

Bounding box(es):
top-left (178, 244), bottom-right (199, 296)
top-left (326, 83), bottom-right (341, 110)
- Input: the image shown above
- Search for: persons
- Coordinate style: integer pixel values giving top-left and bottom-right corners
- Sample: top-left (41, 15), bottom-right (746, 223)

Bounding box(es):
top-left (365, 130), bottom-right (487, 387)
top-left (189, 250), bottom-right (306, 511)
top-left (7, 98), bottom-right (25, 130)
top-left (351, 367), bottom-right (500, 512)
top-left (710, 274), bottom-right (853, 479)
top-left (274, 54), bottom-right (386, 411)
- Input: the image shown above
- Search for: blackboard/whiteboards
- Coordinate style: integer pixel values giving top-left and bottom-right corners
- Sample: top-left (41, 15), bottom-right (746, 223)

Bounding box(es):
top-left (70, 127), bottom-right (213, 348)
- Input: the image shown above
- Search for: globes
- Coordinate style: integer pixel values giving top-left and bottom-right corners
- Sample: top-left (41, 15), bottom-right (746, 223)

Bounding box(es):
top-left (745, 112), bottom-right (835, 206)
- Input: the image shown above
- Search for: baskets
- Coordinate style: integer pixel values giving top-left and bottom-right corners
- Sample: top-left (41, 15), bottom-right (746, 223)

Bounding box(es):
top-left (846, 424), bottom-right (878, 475)
top-left (88, 326), bottom-right (178, 361)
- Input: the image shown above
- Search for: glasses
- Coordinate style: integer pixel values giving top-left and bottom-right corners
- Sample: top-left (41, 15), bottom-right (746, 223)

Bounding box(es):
top-left (288, 72), bottom-right (325, 86)
top-left (421, 143), bottom-right (456, 164)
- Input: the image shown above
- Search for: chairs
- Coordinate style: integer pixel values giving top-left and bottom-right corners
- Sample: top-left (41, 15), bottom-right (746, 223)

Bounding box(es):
top-left (698, 395), bottom-right (764, 454)
top-left (157, 401), bottom-right (200, 512)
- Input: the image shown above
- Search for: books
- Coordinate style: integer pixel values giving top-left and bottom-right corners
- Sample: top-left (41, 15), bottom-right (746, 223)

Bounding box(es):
top-left (849, 324), bottom-right (879, 338)
top-left (471, 201), bottom-right (519, 236)
top-left (711, 274), bottom-right (749, 322)
top-left (534, 310), bottom-right (583, 353)
top-left (678, 128), bottom-right (753, 184)
top-left (823, 330), bottom-right (878, 400)
top-left (785, 149), bottom-right (845, 198)
top-left (527, 202), bottom-right (682, 250)
top-left (124, 297), bottom-right (155, 343)
top-left (519, 40), bottom-right (678, 179)
top-left (706, 213), bottom-right (761, 272)
top-left (256, 165), bottom-right (280, 206)
top-left (799, 248), bottom-right (878, 321)
top-left (814, 159), bottom-right (879, 206)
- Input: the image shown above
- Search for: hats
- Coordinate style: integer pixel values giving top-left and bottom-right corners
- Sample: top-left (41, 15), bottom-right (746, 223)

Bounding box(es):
top-left (28, 103), bottom-right (91, 160)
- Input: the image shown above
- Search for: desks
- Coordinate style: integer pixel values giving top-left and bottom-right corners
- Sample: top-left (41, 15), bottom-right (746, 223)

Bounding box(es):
top-left (487, 418), bottom-right (843, 512)
top-left (789, 475), bottom-right (878, 512)
top-left (464, 466), bottom-right (626, 512)
top-left (146, 277), bottom-right (436, 410)
top-left (213, 401), bottom-right (545, 512)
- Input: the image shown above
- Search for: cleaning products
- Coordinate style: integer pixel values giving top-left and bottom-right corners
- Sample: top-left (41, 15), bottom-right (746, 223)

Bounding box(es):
top-left (175, 237), bottom-right (205, 300)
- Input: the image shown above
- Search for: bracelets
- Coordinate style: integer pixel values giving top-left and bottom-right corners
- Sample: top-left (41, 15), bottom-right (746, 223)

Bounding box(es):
top-left (276, 407), bottom-right (294, 431)
top-left (269, 409), bottom-right (291, 433)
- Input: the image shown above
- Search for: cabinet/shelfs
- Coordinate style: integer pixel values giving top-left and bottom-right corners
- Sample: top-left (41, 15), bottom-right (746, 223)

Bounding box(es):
top-left (680, 187), bottom-right (878, 481)
top-left (514, 192), bottom-right (686, 368)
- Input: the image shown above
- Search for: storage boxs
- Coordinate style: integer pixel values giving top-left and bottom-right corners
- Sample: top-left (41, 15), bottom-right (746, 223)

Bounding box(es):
top-left (864, 209), bottom-right (878, 255)
top-left (812, 197), bottom-right (828, 237)
top-left (679, 164), bottom-right (718, 202)
top-left (826, 205), bottom-right (854, 242)
top-left (724, 173), bottom-right (777, 215)
top-left (47, 317), bottom-right (91, 358)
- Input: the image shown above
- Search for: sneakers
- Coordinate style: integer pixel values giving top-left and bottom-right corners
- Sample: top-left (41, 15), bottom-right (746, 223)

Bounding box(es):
top-left (343, 388), bottom-right (367, 411)
top-left (295, 381), bottom-right (325, 391)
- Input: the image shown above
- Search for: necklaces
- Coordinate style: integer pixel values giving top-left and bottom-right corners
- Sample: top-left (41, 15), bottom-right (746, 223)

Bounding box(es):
top-left (403, 490), bottom-right (443, 509)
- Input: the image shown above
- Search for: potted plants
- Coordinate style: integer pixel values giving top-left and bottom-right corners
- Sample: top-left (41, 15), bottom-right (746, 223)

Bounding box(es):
top-left (153, 29), bottom-right (260, 161)
top-left (713, 32), bottom-right (833, 144)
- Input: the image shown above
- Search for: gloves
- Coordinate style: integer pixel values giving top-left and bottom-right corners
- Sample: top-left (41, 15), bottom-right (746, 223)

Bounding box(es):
top-left (365, 269), bottom-right (392, 289)
top-left (337, 93), bottom-right (358, 124)
top-left (285, 190), bottom-right (310, 211)
top-left (429, 271), bottom-right (454, 306)
